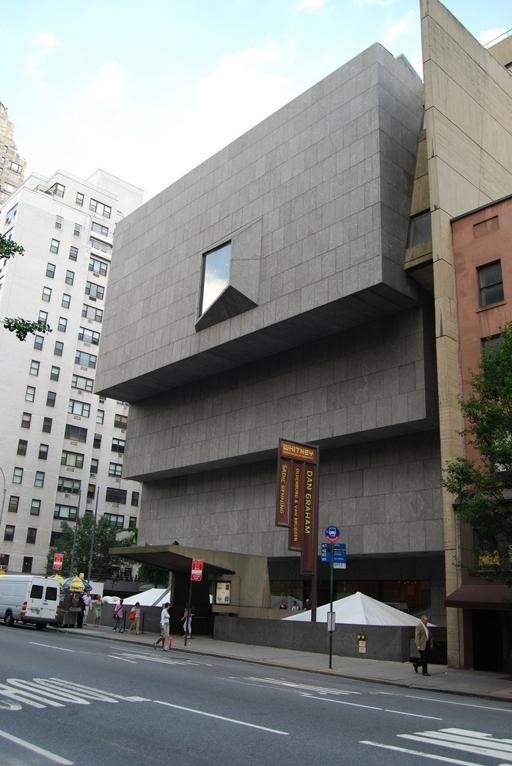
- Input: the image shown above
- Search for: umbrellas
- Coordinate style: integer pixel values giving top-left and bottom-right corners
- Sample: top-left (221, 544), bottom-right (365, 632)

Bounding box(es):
top-left (62, 574), bottom-right (92, 598)
top-left (49, 574), bottom-right (66, 587)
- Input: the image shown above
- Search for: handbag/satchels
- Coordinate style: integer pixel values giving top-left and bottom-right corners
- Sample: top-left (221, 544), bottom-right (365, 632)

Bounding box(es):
top-left (128, 611), bottom-right (135, 620)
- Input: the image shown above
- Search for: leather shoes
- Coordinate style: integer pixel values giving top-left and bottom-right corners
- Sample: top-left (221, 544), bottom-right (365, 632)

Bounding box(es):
top-left (413, 663), bottom-right (418, 673)
top-left (422, 672), bottom-right (431, 676)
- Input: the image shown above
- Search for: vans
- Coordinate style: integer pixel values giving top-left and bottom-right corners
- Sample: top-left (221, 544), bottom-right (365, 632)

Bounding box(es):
top-left (0, 574), bottom-right (61, 631)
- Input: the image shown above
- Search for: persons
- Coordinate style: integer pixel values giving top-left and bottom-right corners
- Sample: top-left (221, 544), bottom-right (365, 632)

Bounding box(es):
top-left (94, 594), bottom-right (103, 624)
top-left (181, 603), bottom-right (195, 639)
top-left (154, 601), bottom-right (172, 649)
top-left (413, 614), bottom-right (432, 677)
top-left (129, 601), bottom-right (142, 635)
top-left (78, 597), bottom-right (86, 629)
top-left (82, 589), bottom-right (93, 625)
top-left (292, 599), bottom-right (300, 610)
top-left (112, 598), bottom-right (125, 633)
top-left (280, 599), bottom-right (288, 609)
